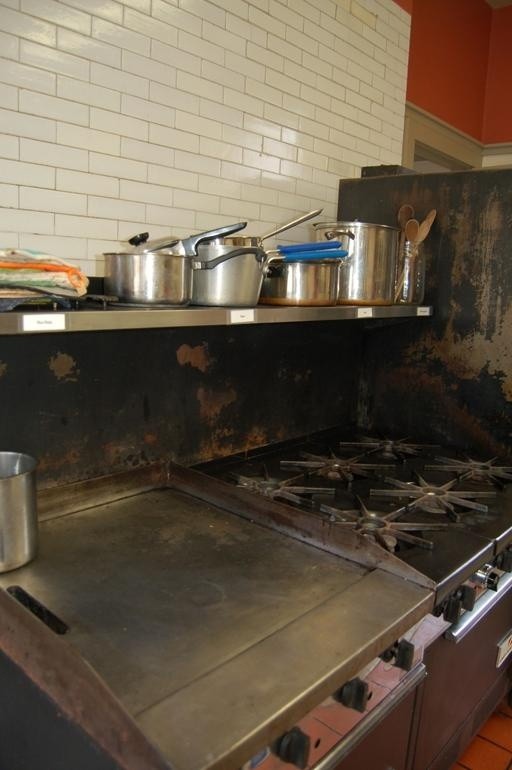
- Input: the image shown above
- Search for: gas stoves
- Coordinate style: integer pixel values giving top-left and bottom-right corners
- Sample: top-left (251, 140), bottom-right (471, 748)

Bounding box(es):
top-left (188, 424), bottom-right (512, 619)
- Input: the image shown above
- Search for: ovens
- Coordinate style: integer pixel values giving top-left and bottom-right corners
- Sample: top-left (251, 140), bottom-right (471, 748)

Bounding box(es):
top-left (405, 571), bottom-right (512, 770)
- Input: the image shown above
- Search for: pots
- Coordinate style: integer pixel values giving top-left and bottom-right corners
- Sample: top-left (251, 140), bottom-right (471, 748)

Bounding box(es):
top-left (312, 217), bottom-right (403, 306)
top-left (259, 261), bottom-right (342, 305)
top-left (102, 208), bottom-right (349, 308)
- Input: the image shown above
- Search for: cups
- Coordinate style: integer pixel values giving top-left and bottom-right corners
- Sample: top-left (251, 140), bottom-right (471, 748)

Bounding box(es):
top-left (395, 241), bottom-right (426, 304)
top-left (0, 451), bottom-right (40, 574)
